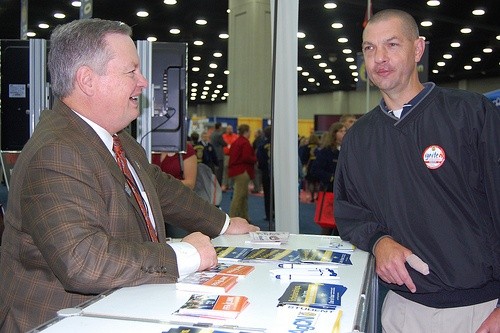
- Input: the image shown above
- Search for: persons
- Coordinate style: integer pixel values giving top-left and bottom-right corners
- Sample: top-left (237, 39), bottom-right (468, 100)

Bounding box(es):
top-left (331, 7), bottom-right (500, 333)
top-left (145, 114), bottom-right (368, 247)
top-left (0, 16), bottom-right (262, 333)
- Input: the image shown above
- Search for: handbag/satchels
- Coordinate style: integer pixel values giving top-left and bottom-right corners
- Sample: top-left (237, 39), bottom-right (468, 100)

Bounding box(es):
top-left (180, 153), bottom-right (222, 206)
top-left (314, 183), bottom-right (337, 229)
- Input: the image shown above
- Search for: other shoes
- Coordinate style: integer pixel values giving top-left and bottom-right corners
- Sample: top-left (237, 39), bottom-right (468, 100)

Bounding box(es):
top-left (221, 184), bottom-right (226, 192)
top-left (264, 215), bottom-right (269, 220)
top-left (251, 188), bottom-right (259, 193)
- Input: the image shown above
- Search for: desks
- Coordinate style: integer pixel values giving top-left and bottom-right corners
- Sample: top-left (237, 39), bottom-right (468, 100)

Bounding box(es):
top-left (27, 233), bottom-right (379, 333)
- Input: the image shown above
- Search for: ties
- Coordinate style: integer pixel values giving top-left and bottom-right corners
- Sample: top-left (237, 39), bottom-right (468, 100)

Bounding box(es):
top-left (112, 135), bottom-right (158, 243)
